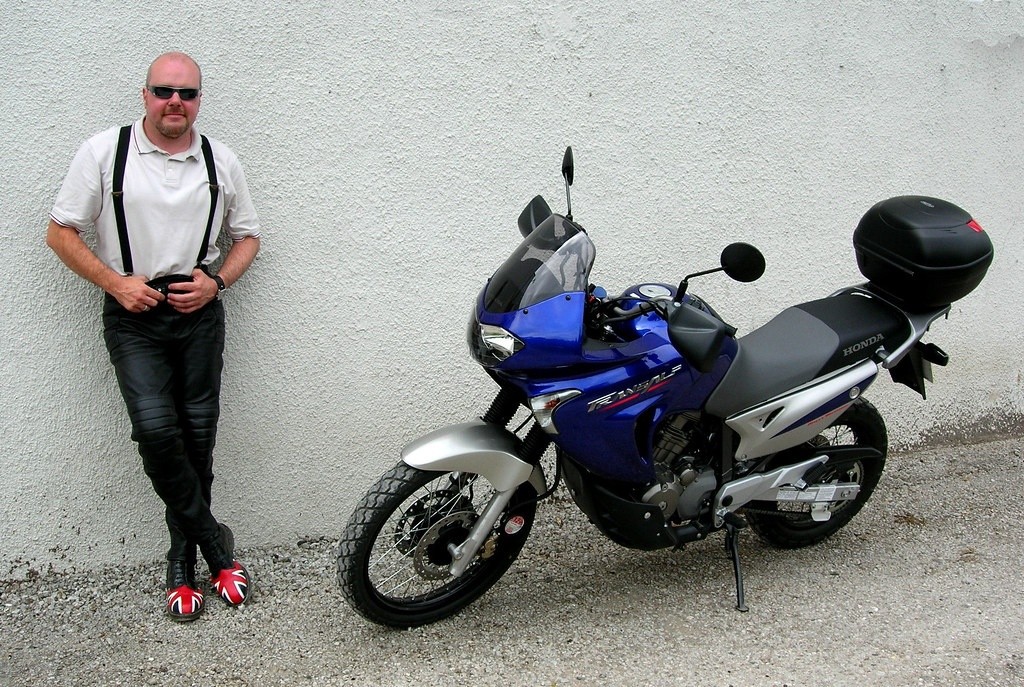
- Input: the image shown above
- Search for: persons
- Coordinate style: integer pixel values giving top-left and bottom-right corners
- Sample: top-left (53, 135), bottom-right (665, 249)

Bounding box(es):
top-left (46, 52), bottom-right (261, 621)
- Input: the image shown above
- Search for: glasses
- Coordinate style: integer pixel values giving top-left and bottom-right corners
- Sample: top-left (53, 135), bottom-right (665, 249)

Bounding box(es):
top-left (146, 86), bottom-right (200, 99)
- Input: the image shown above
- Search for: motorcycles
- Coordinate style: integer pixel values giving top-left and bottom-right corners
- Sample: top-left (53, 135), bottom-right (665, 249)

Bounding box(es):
top-left (333, 144), bottom-right (994, 631)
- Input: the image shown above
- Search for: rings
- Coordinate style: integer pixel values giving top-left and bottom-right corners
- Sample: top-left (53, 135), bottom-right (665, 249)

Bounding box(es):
top-left (143, 305), bottom-right (147, 311)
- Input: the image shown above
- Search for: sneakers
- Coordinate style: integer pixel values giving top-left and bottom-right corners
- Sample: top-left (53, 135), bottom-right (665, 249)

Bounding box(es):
top-left (165, 559), bottom-right (203, 622)
top-left (199, 523), bottom-right (250, 605)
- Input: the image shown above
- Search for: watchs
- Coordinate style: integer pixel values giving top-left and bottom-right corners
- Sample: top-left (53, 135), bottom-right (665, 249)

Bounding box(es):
top-left (213, 276), bottom-right (226, 301)
